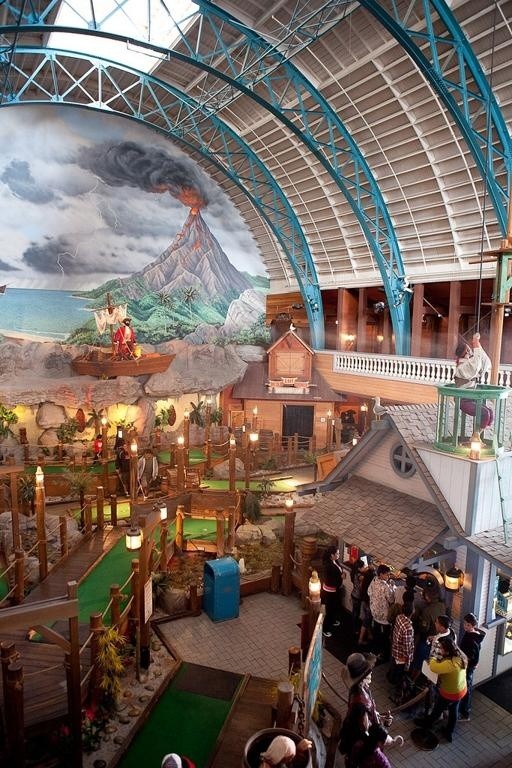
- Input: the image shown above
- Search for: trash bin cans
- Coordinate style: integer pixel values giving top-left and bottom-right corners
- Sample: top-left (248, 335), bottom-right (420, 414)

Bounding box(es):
top-left (204, 556), bottom-right (240, 624)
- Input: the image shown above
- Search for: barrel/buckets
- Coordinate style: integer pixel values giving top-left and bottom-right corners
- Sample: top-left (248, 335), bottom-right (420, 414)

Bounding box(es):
top-left (242, 728), bottom-right (312, 768)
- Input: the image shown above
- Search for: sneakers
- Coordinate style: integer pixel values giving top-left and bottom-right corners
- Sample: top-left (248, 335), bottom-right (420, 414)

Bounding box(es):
top-left (414, 718), bottom-right (432, 728)
top-left (333, 620), bottom-right (340, 626)
top-left (441, 726), bottom-right (452, 742)
top-left (322, 632), bottom-right (332, 637)
top-left (456, 708), bottom-right (471, 722)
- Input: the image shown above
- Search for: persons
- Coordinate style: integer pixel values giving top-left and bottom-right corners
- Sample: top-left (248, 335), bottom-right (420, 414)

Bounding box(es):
top-left (112, 317), bottom-right (138, 362)
top-left (321, 545), bottom-right (487, 768)
top-left (454, 332), bottom-right (495, 449)
top-left (160, 751), bottom-right (195, 768)
top-left (81, 434), bottom-right (159, 499)
top-left (257, 735), bottom-right (314, 768)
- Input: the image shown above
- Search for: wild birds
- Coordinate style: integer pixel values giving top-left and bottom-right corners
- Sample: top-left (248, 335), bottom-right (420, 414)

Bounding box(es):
top-left (372, 395), bottom-right (388, 422)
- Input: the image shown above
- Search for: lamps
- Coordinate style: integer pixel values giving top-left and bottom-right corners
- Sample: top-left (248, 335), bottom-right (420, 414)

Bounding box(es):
top-left (445, 562), bottom-right (464, 594)
top-left (470, 432), bottom-right (483, 460)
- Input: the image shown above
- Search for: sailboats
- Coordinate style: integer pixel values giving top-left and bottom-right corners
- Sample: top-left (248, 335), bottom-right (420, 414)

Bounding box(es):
top-left (70, 292), bottom-right (176, 377)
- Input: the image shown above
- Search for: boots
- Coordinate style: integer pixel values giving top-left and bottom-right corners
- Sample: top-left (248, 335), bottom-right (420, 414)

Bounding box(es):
top-left (471, 428), bottom-right (487, 446)
